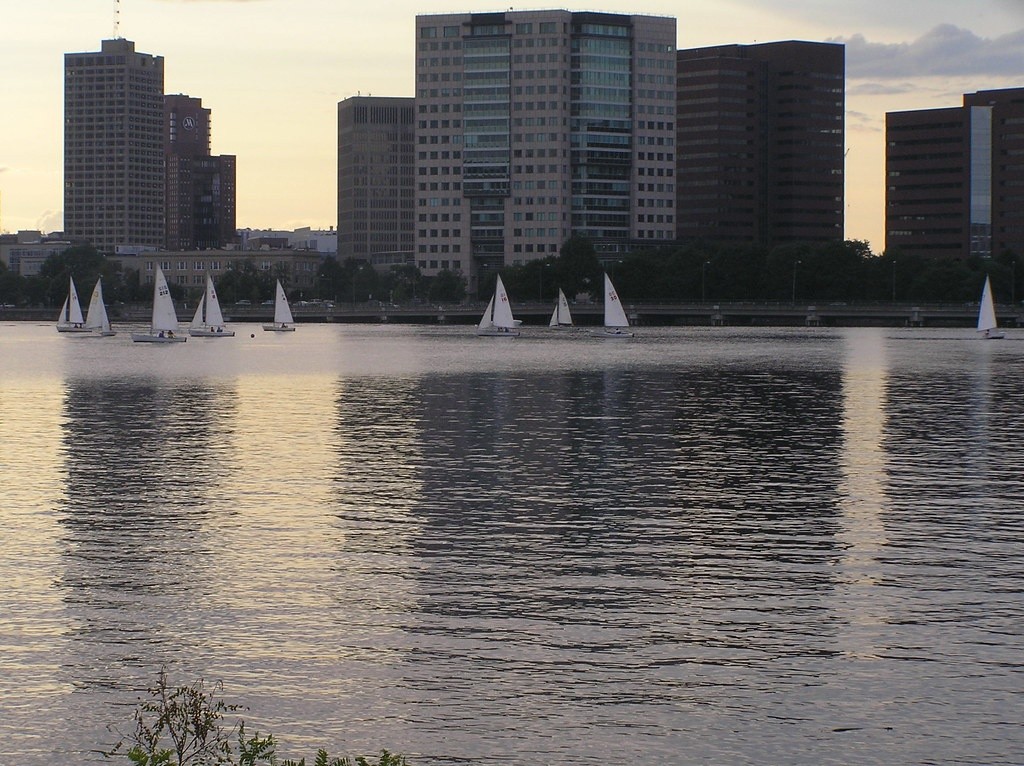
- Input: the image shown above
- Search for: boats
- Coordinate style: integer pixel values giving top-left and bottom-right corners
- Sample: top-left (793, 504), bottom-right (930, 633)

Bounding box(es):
top-left (490, 319), bottom-right (522, 327)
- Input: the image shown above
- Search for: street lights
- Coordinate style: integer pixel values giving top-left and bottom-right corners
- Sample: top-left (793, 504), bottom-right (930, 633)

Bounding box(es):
top-left (892, 260), bottom-right (895, 302)
top-left (703, 261), bottom-right (710, 301)
top-left (352, 266), bottom-right (363, 308)
top-left (792, 261), bottom-right (802, 304)
top-left (1010, 260), bottom-right (1015, 304)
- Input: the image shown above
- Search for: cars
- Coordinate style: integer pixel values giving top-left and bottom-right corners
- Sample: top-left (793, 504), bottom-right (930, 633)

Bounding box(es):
top-left (261, 298), bottom-right (275, 306)
top-left (294, 300), bottom-right (309, 308)
top-left (235, 300), bottom-right (251, 306)
top-left (311, 298), bottom-right (322, 306)
top-left (319, 300), bottom-right (336, 309)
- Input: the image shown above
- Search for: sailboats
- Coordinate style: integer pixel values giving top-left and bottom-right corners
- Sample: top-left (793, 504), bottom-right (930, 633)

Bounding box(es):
top-left (85, 276), bottom-right (117, 336)
top-left (976, 274), bottom-right (1007, 339)
top-left (548, 287), bottom-right (575, 331)
top-left (478, 273), bottom-right (520, 336)
top-left (261, 279), bottom-right (296, 332)
top-left (590, 272), bottom-right (635, 338)
top-left (57, 274), bottom-right (93, 333)
top-left (131, 262), bottom-right (187, 342)
top-left (188, 269), bottom-right (236, 337)
